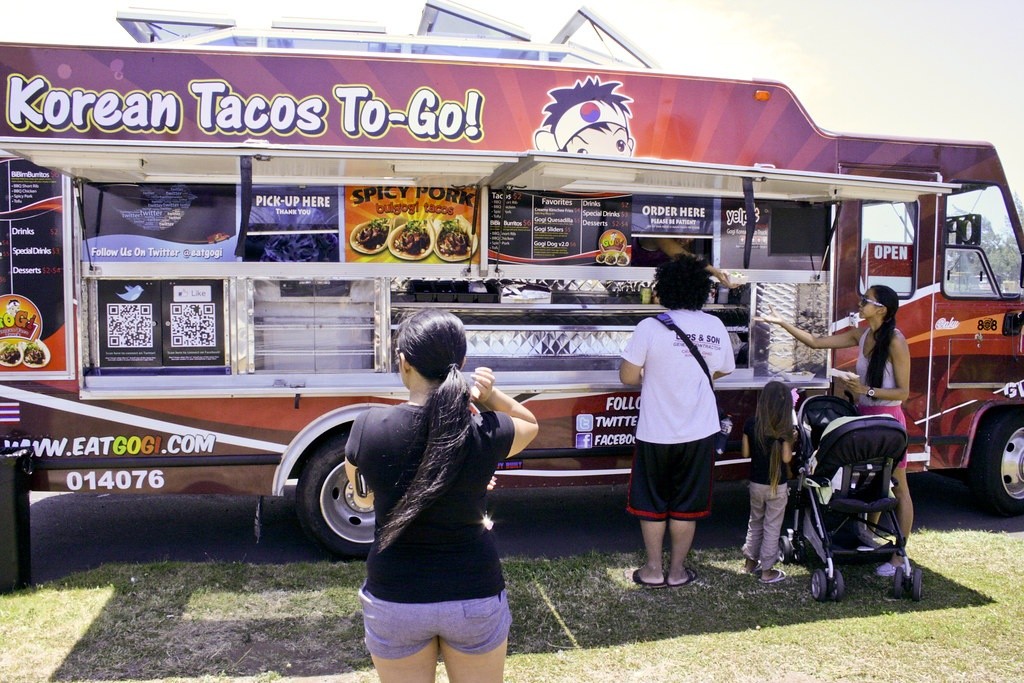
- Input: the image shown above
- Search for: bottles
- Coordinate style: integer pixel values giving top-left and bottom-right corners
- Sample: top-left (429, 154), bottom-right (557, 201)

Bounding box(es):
top-left (705, 282), bottom-right (716, 304)
top-left (716, 414), bottom-right (733, 454)
top-left (718, 282), bottom-right (729, 303)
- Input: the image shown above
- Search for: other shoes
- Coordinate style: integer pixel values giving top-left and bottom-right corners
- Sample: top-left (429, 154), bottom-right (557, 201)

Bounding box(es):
top-left (875, 562), bottom-right (907, 576)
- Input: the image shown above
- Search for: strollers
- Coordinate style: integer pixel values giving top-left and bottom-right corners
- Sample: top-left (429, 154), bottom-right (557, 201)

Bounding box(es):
top-left (777, 385), bottom-right (924, 601)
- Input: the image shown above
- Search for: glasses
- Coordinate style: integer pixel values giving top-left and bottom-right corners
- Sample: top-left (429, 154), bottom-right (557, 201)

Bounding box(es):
top-left (860, 296), bottom-right (884, 307)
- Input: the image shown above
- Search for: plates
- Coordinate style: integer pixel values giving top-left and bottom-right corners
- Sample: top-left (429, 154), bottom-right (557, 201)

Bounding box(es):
top-left (617, 253), bottom-right (629, 266)
top-left (22, 339), bottom-right (50, 368)
top-left (388, 219), bottom-right (434, 260)
top-left (596, 254), bottom-right (606, 264)
top-left (784, 371), bottom-right (814, 382)
top-left (0, 345), bottom-right (23, 367)
top-left (349, 219), bottom-right (392, 255)
top-left (433, 215), bottom-right (478, 262)
top-left (605, 254), bottom-right (617, 265)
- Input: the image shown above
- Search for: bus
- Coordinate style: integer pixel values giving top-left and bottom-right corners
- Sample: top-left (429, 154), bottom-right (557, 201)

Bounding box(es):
top-left (1, 40), bottom-right (1024, 520)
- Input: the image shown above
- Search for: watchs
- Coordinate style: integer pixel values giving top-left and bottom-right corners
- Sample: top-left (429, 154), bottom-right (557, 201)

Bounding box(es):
top-left (867, 386), bottom-right (874, 398)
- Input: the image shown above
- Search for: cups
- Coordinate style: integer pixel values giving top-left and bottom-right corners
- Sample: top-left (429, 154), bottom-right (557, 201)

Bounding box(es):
top-left (641, 287), bottom-right (652, 304)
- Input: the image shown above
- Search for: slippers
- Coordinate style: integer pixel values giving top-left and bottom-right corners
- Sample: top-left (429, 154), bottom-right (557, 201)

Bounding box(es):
top-left (758, 569), bottom-right (787, 584)
top-left (667, 568), bottom-right (697, 588)
top-left (741, 559), bottom-right (762, 574)
top-left (624, 568), bottom-right (667, 588)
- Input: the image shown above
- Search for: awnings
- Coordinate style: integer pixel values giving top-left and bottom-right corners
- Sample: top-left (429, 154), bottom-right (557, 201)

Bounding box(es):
top-left (0, 136), bottom-right (963, 203)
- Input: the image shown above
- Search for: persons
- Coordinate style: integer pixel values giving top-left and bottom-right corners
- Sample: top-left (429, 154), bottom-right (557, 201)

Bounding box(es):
top-left (620, 254), bottom-right (735, 588)
top-left (632, 237), bottom-right (731, 288)
top-left (750, 285), bottom-right (913, 576)
top-left (345, 309), bottom-right (538, 683)
top-left (742, 380), bottom-right (798, 582)
